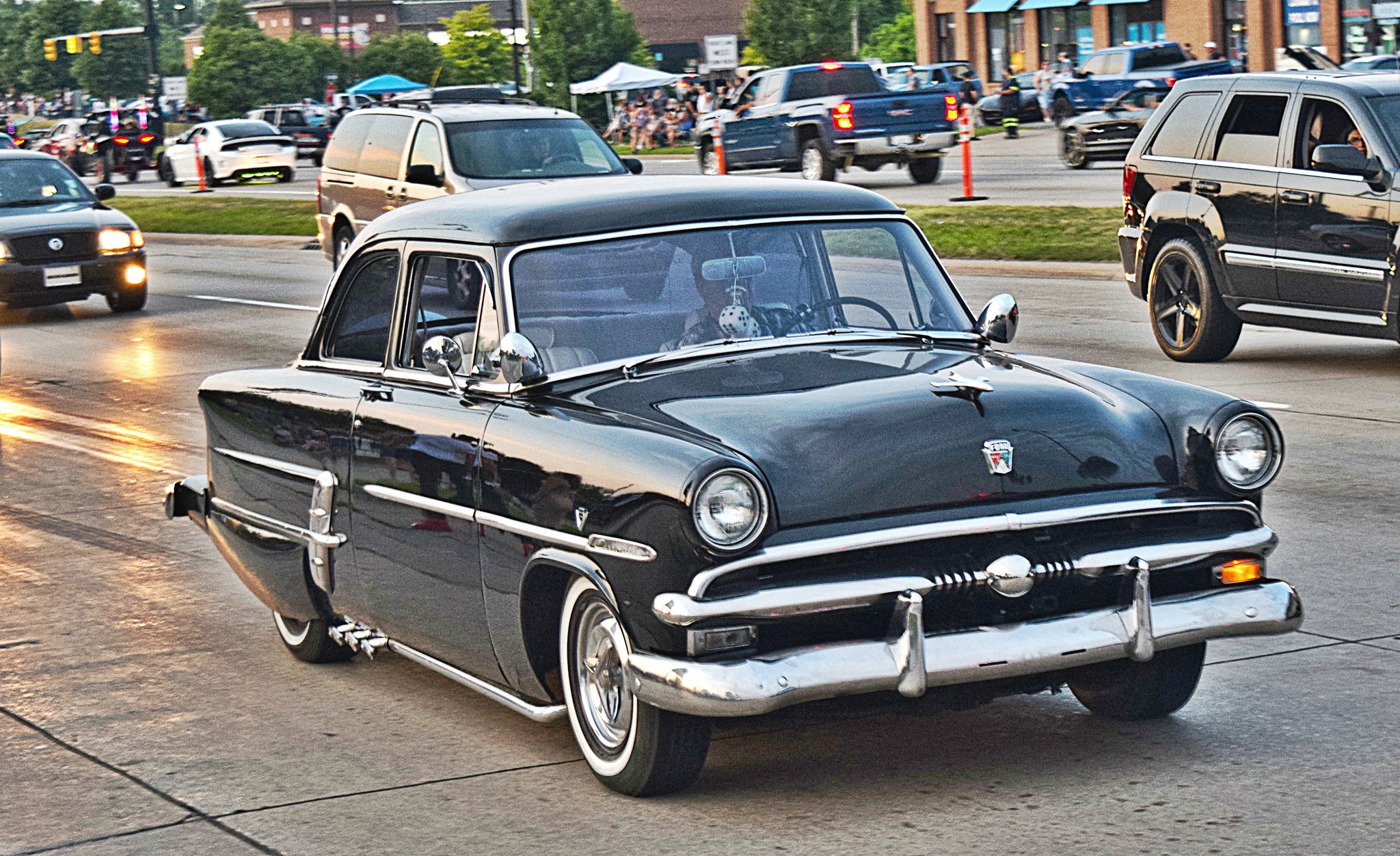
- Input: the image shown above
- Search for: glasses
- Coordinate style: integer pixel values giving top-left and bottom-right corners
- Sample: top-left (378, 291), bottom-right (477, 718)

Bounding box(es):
top-left (1347, 137), bottom-right (1365, 144)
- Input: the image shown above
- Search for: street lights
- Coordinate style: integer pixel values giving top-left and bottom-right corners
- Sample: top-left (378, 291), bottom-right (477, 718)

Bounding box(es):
top-left (501, 2), bottom-right (527, 95)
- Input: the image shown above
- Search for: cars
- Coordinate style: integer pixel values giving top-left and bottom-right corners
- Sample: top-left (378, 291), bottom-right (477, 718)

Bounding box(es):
top-left (162, 175), bottom-right (1303, 797)
top-left (157, 117), bottom-right (295, 187)
top-left (0, 96), bottom-right (211, 315)
top-left (873, 41), bottom-right (1400, 171)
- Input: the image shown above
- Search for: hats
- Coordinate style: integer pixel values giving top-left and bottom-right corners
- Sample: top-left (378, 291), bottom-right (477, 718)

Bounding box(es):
top-left (1203, 42), bottom-right (1217, 48)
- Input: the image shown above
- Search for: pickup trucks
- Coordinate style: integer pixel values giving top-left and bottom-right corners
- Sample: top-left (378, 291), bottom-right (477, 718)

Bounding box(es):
top-left (240, 84), bottom-right (538, 167)
top-left (693, 61), bottom-right (959, 183)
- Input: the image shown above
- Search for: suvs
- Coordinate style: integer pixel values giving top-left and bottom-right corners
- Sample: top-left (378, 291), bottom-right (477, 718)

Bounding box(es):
top-left (1116, 71), bottom-right (1400, 365)
top-left (315, 97), bottom-right (674, 310)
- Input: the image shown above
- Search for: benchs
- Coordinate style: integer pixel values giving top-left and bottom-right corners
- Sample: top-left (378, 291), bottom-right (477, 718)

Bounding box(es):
top-left (339, 293), bottom-right (691, 378)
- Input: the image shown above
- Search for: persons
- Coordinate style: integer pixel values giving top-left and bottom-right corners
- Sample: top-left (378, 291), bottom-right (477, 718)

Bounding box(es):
top-left (1033, 60), bottom-right (1056, 123)
top-left (735, 92), bottom-right (757, 114)
top-left (602, 77), bottom-right (749, 152)
top-left (1058, 53), bottom-right (1074, 73)
top-left (905, 68), bottom-right (919, 90)
top-left (1204, 42), bottom-right (1226, 61)
top-left (997, 67), bottom-right (1021, 139)
top-left (677, 231), bottom-right (813, 347)
top-left (329, 99), bottom-right (353, 117)
top-left (1346, 130), bottom-right (1368, 160)
top-left (960, 70), bottom-right (981, 141)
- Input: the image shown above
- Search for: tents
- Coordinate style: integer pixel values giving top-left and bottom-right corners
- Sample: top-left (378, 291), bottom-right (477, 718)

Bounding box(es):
top-left (569, 61), bottom-right (700, 143)
top-left (347, 74), bottom-right (429, 106)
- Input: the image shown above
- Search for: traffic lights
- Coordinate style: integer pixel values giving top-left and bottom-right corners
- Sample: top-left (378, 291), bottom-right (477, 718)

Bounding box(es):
top-left (43, 39), bottom-right (57, 62)
top-left (88, 30), bottom-right (100, 53)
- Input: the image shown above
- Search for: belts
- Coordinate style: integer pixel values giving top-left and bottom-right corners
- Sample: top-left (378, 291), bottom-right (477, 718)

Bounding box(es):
top-left (699, 112), bottom-right (709, 115)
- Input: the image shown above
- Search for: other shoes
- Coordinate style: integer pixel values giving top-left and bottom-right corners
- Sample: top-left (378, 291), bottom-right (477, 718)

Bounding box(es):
top-left (1043, 117), bottom-right (1052, 123)
top-left (970, 137), bottom-right (980, 141)
top-left (1003, 135), bottom-right (1018, 139)
top-left (606, 141), bottom-right (701, 155)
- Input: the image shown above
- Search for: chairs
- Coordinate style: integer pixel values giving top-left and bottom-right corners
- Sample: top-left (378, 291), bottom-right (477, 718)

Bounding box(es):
top-left (1308, 112), bottom-right (1351, 168)
top-left (451, 327), bottom-right (599, 384)
